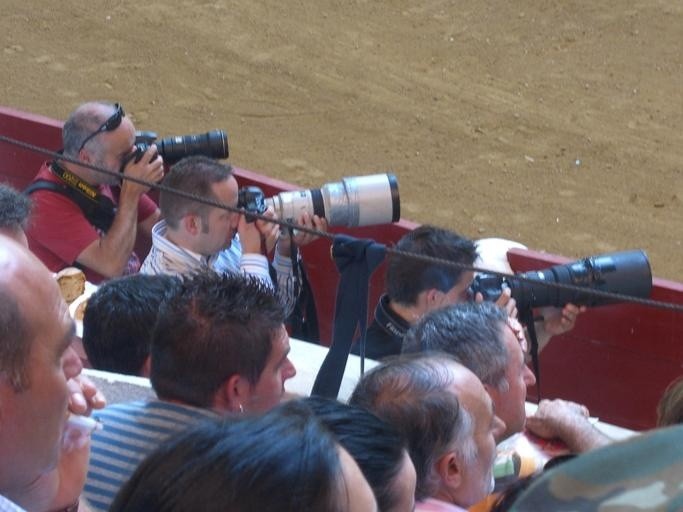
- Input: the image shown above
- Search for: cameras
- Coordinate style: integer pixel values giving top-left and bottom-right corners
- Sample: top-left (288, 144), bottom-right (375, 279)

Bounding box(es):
top-left (130, 124), bottom-right (231, 185)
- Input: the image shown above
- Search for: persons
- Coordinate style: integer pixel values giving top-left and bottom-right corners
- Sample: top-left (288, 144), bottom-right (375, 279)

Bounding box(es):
top-left (133, 154), bottom-right (330, 327)
top-left (292, 394), bottom-right (419, 512)
top-left (346, 347), bottom-right (510, 511)
top-left (0, 178), bottom-right (38, 254)
top-left (16, 101), bottom-right (162, 288)
top-left (400, 297), bottom-right (622, 510)
top-left (79, 272), bottom-right (187, 380)
top-left (0, 229), bottom-right (108, 512)
top-left (346, 223), bottom-right (588, 369)
top-left (106, 402), bottom-right (380, 512)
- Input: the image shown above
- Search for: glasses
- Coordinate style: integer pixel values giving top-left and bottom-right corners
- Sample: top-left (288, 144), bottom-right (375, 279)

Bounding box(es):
top-left (78, 102), bottom-right (125, 150)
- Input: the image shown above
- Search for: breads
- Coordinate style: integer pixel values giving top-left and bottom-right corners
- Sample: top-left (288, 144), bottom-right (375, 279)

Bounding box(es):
top-left (55, 266), bottom-right (86, 302)
top-left (74, 300), bottom-right (87, 318)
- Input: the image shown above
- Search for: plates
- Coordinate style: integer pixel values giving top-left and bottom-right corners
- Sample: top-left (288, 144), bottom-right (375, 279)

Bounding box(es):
top-left (67, 291), bottom-right (94, 339)
